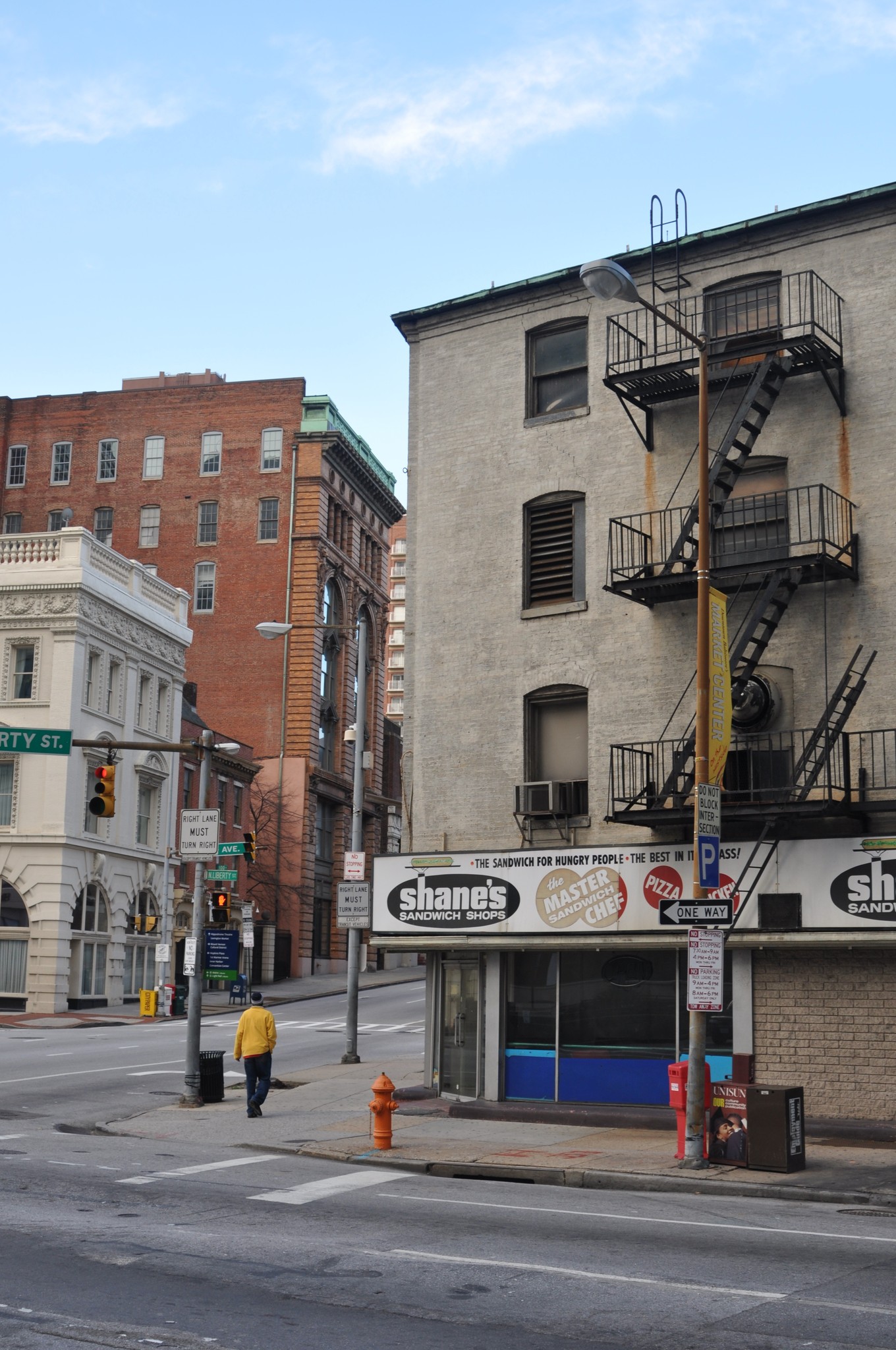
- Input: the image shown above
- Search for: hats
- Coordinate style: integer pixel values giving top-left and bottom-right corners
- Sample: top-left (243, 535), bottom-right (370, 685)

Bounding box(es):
top-left (251, 993), bottom-right (263, 1004)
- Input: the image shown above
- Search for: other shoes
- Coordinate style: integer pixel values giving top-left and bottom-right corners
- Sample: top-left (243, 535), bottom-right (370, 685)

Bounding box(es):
top-left (250, 1100), bottom-right (262, 1115)
top-left (248, 1112), bottom-right (256, 1117)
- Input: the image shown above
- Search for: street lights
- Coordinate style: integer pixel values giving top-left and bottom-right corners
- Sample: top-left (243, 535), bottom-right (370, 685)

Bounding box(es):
top-left (579, 256), bottom-right (716, 1167)
top-left (156, 742), bottom-right (240, 1018)
top-left (256, 622), bottom-right (364, 1065)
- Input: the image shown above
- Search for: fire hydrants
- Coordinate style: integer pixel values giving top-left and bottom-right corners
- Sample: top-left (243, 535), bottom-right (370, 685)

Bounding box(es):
top-left (366, 1070), bottom-right (399, 1150)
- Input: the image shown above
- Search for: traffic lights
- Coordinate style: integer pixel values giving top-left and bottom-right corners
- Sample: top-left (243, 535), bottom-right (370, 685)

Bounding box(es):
top-left (145, 913), bottom-right (158, 933)
top-left (128, 916), bottom-right (141, 932)
top-left (208, 890), bottom-right (232, 925)
top-left (88, 764), bottom-right (116, 820)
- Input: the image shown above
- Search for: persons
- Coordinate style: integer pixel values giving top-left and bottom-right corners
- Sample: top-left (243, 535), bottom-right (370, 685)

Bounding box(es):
top-left (234, 993), bottom-right (277, 1118)
top-left (726, 1113), bottom-right (746, 1161)
top-left (709, 1106), bottom-right (735, 1158)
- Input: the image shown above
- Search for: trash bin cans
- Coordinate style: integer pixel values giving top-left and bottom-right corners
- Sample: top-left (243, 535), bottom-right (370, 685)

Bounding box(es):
top-left (199, 1051), bottom-right (226, 1103)
top-left (138, 988), bottom-right (158, 1017)
top-left (709, 1081), bottom-right (805, 1173)
top-left (229, 974), bottom-right (247, 999)
top-left (154, 985), bottom-right (187, 1016)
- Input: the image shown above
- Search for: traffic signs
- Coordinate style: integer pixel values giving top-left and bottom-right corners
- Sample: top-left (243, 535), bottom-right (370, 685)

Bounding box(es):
top-left (657, 899), bottom-right (734, 926)
